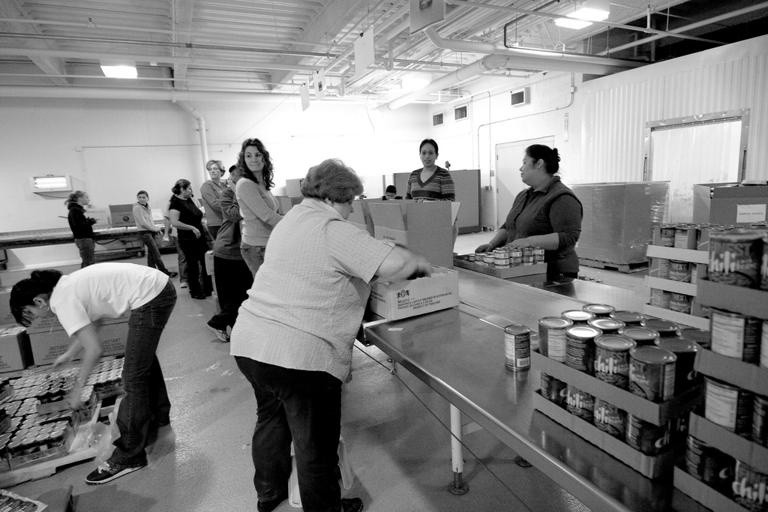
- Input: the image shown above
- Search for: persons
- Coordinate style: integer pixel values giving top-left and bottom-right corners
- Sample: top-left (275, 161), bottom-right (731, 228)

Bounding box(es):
top-left (228, 162), bottom-right (437, 512)
top-left (473, 144), bottom-right (583, 279)
top-left (373, 185), bottom-right (400, 198)
top-left (132, 191), bottom-right (178, 278)
top-left (202, 160), bottom-right (226, 240)
top-left (169, 179), bottom-right (212, 299)
top-left (402, 137), bottom-right (455, 206)
top-left (11, 262), bottom-right (176, 485)
top-left (208, 169), bottom-right (252, 340)
top-left (67, 191), bottom-right (97, 269)
top-left (229, 141), bottom-right (285, 281)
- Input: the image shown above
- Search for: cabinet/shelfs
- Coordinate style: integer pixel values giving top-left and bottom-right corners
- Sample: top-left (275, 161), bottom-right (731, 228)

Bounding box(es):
top-left (530, 344), bottom-right (705, 481)
top-left (642, 219), bottom-right (716, 335)
top-left (675, 279), bottom-right (768, 512)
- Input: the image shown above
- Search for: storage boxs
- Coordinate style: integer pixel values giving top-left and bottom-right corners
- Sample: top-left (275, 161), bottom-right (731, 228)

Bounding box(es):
top-left (372, 268), bottom-right (458, 322)
top-left (692, 182), bottom-right (767, 228)
top-left (369, 200), bottom-right (460, 273)
top-left (0, 326), bottom-right (31, 373)
top-left (572, 182), bottom-right (669, 270)
top-left (109, 204), bottom-right (138, 227)
top-left (27, 310), bottom-right (141, 367)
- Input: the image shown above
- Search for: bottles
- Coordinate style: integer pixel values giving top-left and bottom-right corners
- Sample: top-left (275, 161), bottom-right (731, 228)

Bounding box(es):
top-left (673, 402), bottom-right (704, 437)
top-left (679, 222), bottom-right (734, 249)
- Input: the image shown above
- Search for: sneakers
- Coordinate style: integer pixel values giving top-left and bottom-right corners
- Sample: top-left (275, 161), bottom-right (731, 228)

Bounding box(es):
top-left (86, 461), bottom-right (144, 485)
top-left (205, 323), bottom-right (229, 341)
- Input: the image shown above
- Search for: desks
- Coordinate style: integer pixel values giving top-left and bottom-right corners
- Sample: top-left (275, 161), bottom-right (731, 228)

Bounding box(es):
top-left (360, 266), bottom-right (628, 512)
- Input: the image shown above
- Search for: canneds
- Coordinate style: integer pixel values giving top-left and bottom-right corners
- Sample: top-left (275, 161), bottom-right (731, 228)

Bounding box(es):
top-left (469, 255), bottom-right (476, 261)
top-left (760, 322), bottom-right (768, 368)
top-left (707, 232), bottom-right (763, 286)
top-left (587, 317), bottom-right (626, 333)
top-left (23, 438), bottom-right (39, 454)
top-left (523, 263), bottom-right (534, 266)
top-left (533, 247), bottom-right (544, 256)
top-left (676, 328), bottom-right (712, 349)
top-left (670, 292), bottom-right (691, 314)
top-left (533, 255), bottom-right (544, 261)
top-left (49, 388), bottom-right (63, 402)
top-left (610, 310), bottom-right (644, 326)
top-left (1, 398), bottom-right (40, 409)
top-left (735, 220), bottom-right (768, 237)
top-left (508, 249), bottom-right (522, 258)
top-left (522, 256), bottom-right (533, 263)
top-left (475, 253), bottom-right (488, 261)
top-left (618, 325), bottom-right (660, 345)
top-left (494, 249), bottom-right (510, 259)
top-left (538, 316), bottom-right (573, 363)
top-left (38, 390), bottom-right (48, 403)
top-left (628, 345), bottom-right (677, 403)
top-left (593, 397), bottom-right (626, 440)
top-left (690, 297), bottom-right (710, 318)
top-left (504, 325), bottom-right (531, 372)
top-left (685, 435), bottom-right (734, 496)
top-left (652, 224), bottom-right (676, 247)
top-left (483, 255), bottom-right (495, 264)
top-left (50, 432), bottom-right (64, 449)
top-left (710, 310), bottom-right (761, 365)
top-left (674, 226), bottom-right (698, 249)
top-left (593, 334), bottom-right (636, 391)
top-left (625, 413), bottom-right (671, 456)
top-left (510, 257), bottom-right (522, 264)
top-left (668, 260), bottom-right (691, 283)
top-left (534, 260), bottom-right (544, 265)
top-left (566, 325), bottom-right (603, 377)
top-left (12, 409), bottom-right (80, 442)
top-left (654, 336), bottom-right (698, 396)
top-left (704, 377), bottom-right (753, 438)
top-left (583, 302), bottom-right (615, 318)
top-left (760, 235), bottom-right (768, 290)
top-left (541, 370), bottom-right (567, 409)
top-left (7, 441), bottom-right (22, 457)
top-left (1, 367), bottom-right (79, 397)
top-left (752, 395), bottom-right (768, 447)
top-left (640, 319), bottom-right (678, 337)
top-left (486, 248), bottom-right (501, 255)
top-left (36, 434), bottom-right (50, 450)
top-left (561, 309), bottom-right (596, 326)
top-left (521, 248), bottom-right (533, 256)
top-left (652, 290), bottom-right (671, 308)
top-left (732, 459), bottom-right (768, 511)
top-left (651, 257), bottom-right (669, 279)
top-left (79, 356), bottom-right (125, 421)
top-left (566, 384), bottom-right (594, 424)
top-left (691, 262), bottom-right (707, 284)
top-left (493, 258), bottom-right (510, 266)
top-left (1, 411), bottom-right (13, 456)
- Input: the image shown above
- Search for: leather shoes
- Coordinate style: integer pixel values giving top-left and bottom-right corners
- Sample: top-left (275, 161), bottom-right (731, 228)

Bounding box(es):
top-left (342, 497), bottom-right (363, 511)
top-left (258, 491), bottom-right (287, 511)
